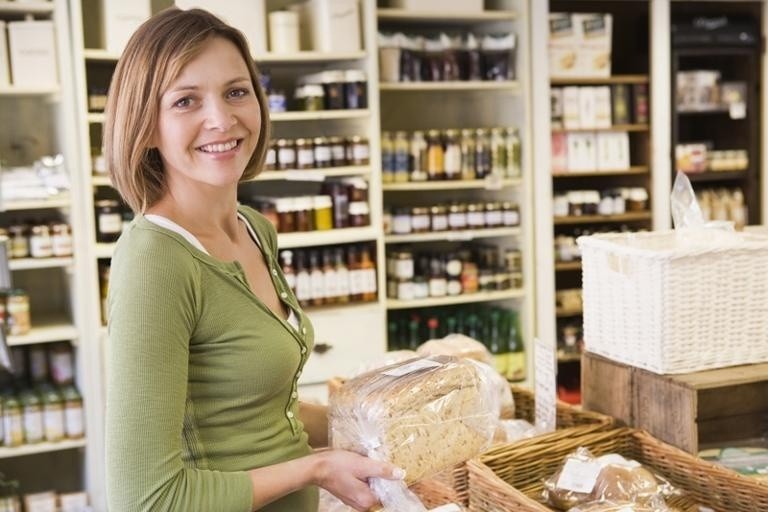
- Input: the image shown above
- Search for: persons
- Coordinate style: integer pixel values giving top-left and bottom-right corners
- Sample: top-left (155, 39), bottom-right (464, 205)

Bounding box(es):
top-left (101, 6), bottom-right (410, 511)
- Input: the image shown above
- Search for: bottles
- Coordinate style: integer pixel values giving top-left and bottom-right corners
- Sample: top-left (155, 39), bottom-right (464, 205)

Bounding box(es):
top-left (381, 125), bottom-right (522, 182)
top-left (281, 248), bottom-right (378, 308)
top-left (387, 304), bottom-right (527, 382)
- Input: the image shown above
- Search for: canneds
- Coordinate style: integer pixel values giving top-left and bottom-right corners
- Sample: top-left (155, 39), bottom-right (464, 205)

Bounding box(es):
top-left (382, 202), bottom-right (521, 235)
top-left (0, 224), bottom-right (84, 448)
top-left (95, 200), bottom-right (124, 242)
top-left (263, 135), bottom-right (369, 172)
top-left (385, 250), bottom-right (428, 299)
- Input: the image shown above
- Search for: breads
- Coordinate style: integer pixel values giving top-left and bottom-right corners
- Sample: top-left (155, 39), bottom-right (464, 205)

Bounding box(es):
top-left (544, 447), bottom-right (670, 512)
top-left (327, 333), bottom-right (515, 486)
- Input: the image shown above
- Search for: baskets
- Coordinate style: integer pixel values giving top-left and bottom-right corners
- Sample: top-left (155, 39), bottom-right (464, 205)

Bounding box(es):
top-left (576, 218), bottom-right (768, 374)
top-left (326, 377), bottom-right (613, 511)
top-left (470, 425), bottom-right (767, 511)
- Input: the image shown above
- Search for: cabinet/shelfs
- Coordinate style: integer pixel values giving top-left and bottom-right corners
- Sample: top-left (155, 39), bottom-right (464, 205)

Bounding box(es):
top-left (1, 0), bottom-right (766, 510)
